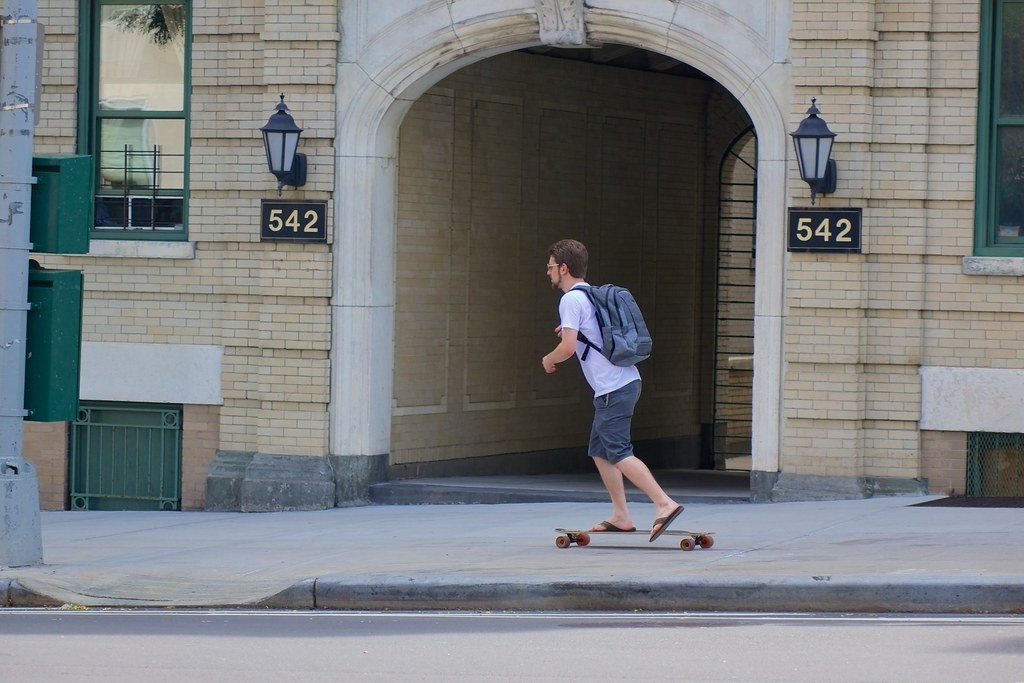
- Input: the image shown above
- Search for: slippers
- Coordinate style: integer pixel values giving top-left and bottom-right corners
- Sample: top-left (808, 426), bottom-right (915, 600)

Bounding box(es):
top-left (589, 521), bottom-right (636, 532)
top-left (649, 505), bottom-right (684, 542)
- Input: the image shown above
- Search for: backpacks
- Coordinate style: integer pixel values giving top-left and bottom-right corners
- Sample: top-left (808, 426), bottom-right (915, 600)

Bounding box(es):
top-left (559, 284), bottom-right (652, 367)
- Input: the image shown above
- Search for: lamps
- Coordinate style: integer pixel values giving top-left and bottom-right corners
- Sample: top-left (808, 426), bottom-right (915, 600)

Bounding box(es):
top-left (259, 91), bottom-right (307, 198)
top-left (789, 96), bottom-right (837, 206)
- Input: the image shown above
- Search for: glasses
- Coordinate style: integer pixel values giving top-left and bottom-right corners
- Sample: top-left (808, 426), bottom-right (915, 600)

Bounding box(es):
top-left (547, 264), bottom-right (560, 269)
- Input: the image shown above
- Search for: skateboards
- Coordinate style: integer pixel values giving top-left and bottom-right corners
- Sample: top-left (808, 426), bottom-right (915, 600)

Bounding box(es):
top-left (554, 528), bottom-right (717, 551)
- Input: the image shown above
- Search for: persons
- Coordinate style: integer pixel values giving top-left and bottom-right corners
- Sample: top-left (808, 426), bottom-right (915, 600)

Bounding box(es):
top-left (540, 239), bottom-right (684, 544)
